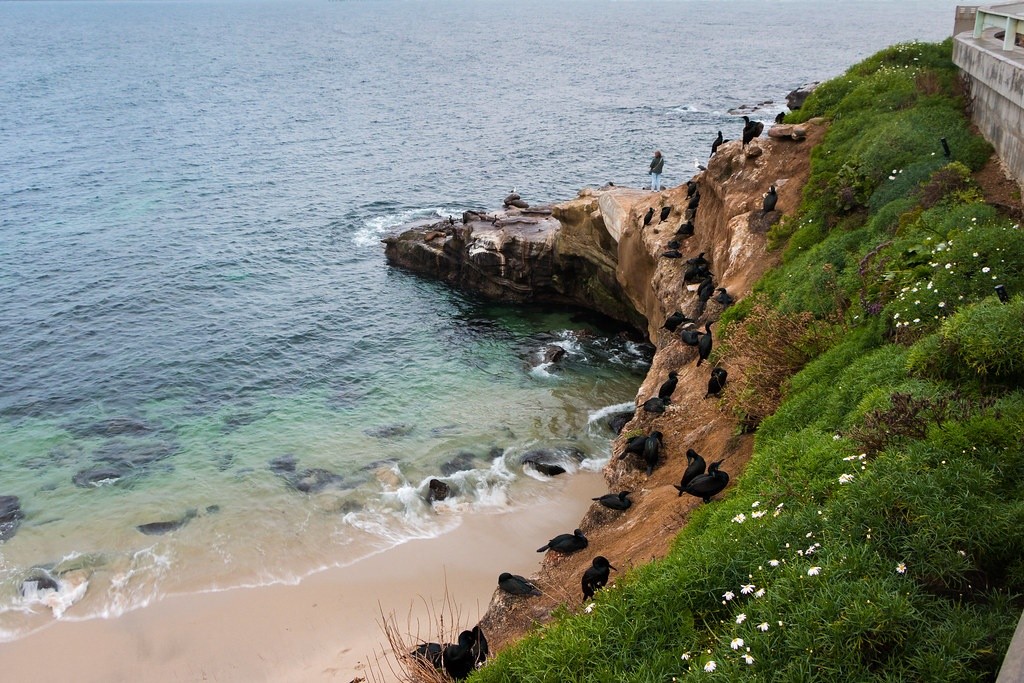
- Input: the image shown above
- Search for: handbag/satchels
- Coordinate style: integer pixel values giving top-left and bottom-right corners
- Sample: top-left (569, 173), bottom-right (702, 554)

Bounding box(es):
top-left (649, 169), bottom-right (652, 175)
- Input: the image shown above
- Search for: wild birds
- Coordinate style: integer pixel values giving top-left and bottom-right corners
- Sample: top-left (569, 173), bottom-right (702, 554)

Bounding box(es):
top-left (399, 624), bottom-right (490, 683)
top-left (759, 185), bottom-right (778, 220)
top-left (681, 252), bottom-right (736, 312)
top-left (636, 371), bottom-right (683, 421)
top-left (673, 449), bottom-right (729, 504)
top-left (641, 180), bottom-right (701, 259)
top-left (693, 157), bottom-right (708, 173)
top-left (701, 367), bottom-right (728, 401)
top-left (498, 572), bottom-right (543, 597)
top-left (617, 430), bottom-right (667, 477)
top-left (709, 131), bottom-right (723, 158)
top-left (581, 556), bottom-right (617, 605)
top-left (591, 491), bottom-right (633, 511)
top-left (739, 116), bottom-right (764, 151)
top-left (775, 111), bottom-right (785, 124)
top-left (537, 528), bottom-right (589, 556)
top-left (659, 311), bottom-right (716, 368)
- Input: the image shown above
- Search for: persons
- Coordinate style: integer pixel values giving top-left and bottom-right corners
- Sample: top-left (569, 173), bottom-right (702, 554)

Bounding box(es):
top-left (649, 150), bottom-right (664, 192)
